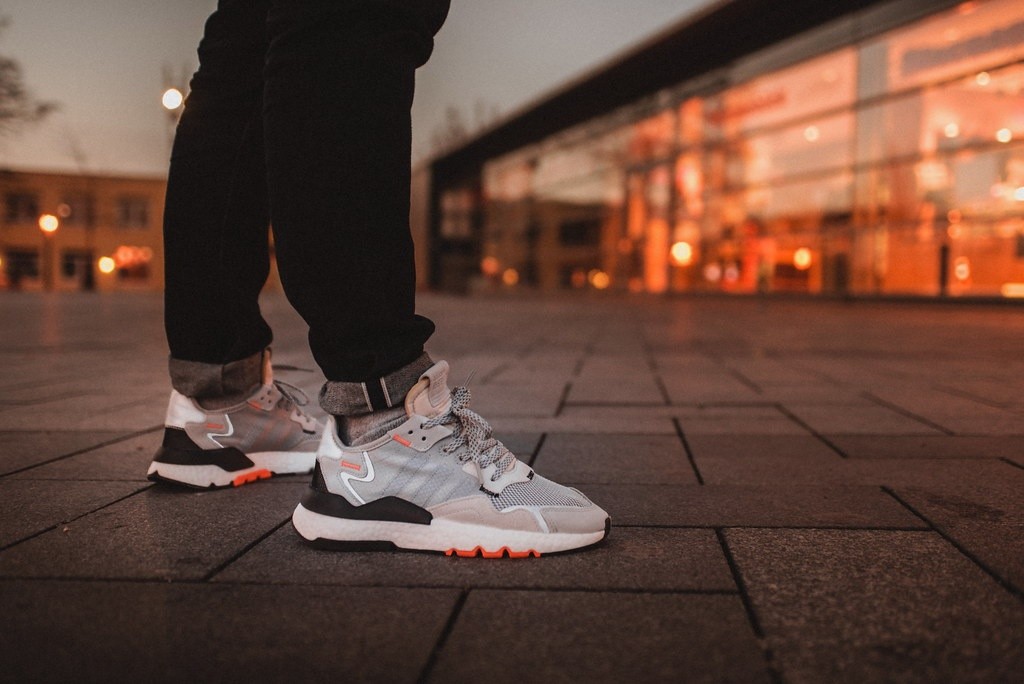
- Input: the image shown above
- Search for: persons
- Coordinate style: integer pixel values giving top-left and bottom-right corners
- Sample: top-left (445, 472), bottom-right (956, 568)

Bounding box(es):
top-left (147, 0), bottom-right (612, 557)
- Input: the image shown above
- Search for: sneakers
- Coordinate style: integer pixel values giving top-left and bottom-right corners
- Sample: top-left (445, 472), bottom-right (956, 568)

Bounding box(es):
top-left (147, 346), bottom-right (324, 486)
top-left (292, 360), bottom-right (610, 559)
